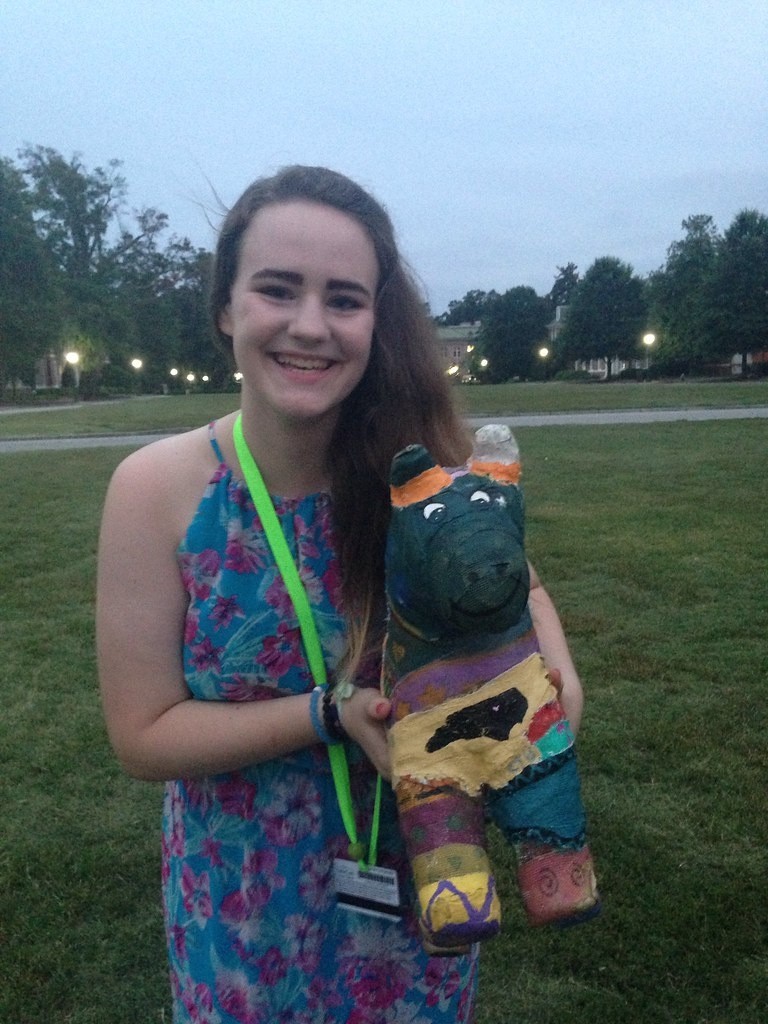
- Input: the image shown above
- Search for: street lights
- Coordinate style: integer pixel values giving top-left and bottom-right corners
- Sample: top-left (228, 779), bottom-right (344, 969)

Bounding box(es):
top-left (133, 361), bottom-right (141, 397)
top-left (540, 347), bottom-right (548, 380)
top-left (642, 332), bottom-right (653, 384)
top-left (171, 367), bottom-right (179, 397)
top-left (66, 350), bottom-right (78, 402)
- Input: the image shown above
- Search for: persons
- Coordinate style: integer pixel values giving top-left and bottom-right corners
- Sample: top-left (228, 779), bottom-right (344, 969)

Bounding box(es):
top-left (95, 165), bottom-right (582, 1024)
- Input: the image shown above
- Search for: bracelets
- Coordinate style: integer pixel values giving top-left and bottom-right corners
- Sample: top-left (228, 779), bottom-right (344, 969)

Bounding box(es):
top-left (310, 683), bottom-right (349, 745)
